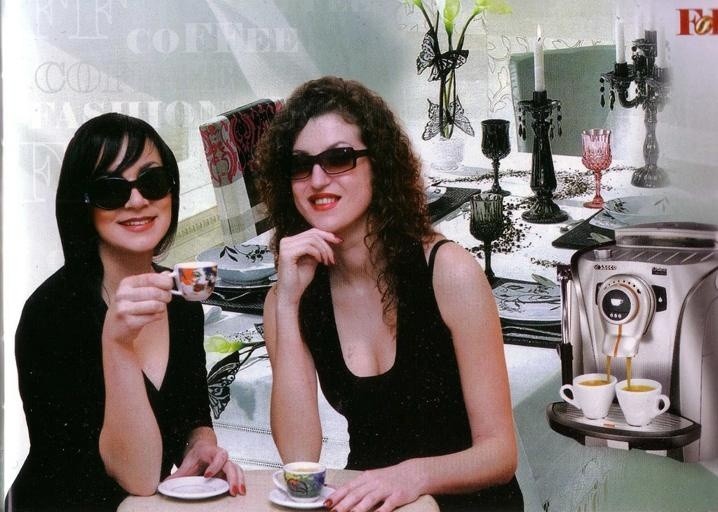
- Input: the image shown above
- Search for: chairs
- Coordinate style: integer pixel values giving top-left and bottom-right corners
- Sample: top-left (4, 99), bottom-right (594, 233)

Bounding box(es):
top-left (197, 95), bottom-right (287, 249)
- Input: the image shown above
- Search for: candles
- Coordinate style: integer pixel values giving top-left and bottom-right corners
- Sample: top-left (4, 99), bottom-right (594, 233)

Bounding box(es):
top-left (610, 1), bottom-right (667, 74)
top-left (532, 20), bottom-right (548, 92)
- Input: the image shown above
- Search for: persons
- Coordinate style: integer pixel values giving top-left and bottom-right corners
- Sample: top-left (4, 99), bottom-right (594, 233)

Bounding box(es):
top-left (6, 111), bottom-right (247, 512)
top-left (262, 75), bottom-right (526, 512)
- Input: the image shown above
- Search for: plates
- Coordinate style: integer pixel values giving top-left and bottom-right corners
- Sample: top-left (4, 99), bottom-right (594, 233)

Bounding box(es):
top-left (419, 186), bottom-right (447, 208)
top-left (602, 192), bottom-right (679, 225)
top-left (158, 476), bottom-right (231, 502)
top-left (268, 487), bottom-right (335, 509)
top-left (195, 245), bottom-right (279, 284)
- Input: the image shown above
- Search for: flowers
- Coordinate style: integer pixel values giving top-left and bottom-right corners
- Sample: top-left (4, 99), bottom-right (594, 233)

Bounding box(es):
top-left (401, 0), bottom-right (516, 142)
top-left (201, 329), bottom-right (268, 379)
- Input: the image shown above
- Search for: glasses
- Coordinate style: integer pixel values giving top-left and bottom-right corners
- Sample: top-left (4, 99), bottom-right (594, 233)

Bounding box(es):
top-left (284, 147), bottom-right (370, 180)
top-left (83, 167), bottom-right (175, 210)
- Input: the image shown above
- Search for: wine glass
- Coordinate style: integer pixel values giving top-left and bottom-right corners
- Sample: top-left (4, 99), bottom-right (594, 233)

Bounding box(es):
top-left (577, 125), bottom-right (613, 213)
top-left (480, 118), bottom-right (514, 197)
top-left (467, 192), bottom-right (508, 285)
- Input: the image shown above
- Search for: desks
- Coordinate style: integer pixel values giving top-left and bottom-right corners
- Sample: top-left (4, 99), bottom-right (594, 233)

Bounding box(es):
top-left (117, 462), bottom-right (443, 512)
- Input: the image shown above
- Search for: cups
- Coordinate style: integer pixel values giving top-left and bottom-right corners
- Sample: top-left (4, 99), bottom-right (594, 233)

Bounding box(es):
top-left (617, 378), bottom-right (670, 428)
top-left (273, 460), bottom-right (325, 504)
top-left (558, 373), bottom-right (617, 419)
top-left (164, 259), bottom-right (216, 302)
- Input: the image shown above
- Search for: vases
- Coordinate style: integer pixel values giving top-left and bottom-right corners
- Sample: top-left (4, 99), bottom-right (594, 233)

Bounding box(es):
top-left (423, 135), bottom-right (466, 174)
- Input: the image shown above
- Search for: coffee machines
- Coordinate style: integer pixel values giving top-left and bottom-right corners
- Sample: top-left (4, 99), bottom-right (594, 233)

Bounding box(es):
top-left (544, 220), bottom-right (718, 464)
